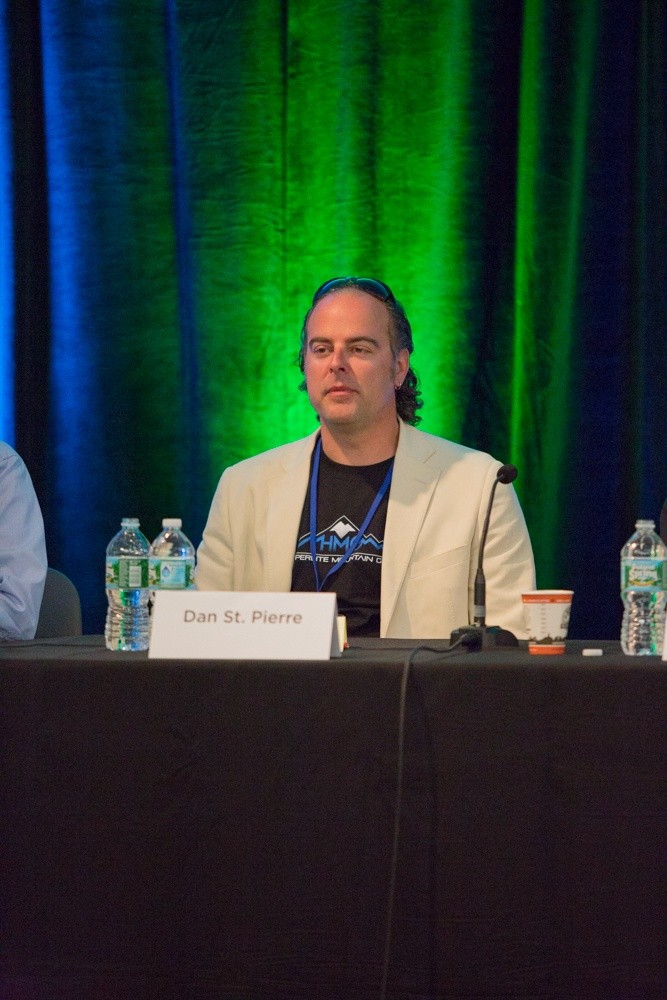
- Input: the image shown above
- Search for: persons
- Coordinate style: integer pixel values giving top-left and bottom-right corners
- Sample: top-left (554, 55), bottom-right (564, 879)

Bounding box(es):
top-left (193, 278), bottom-right (536, 640)
top-left (0, 438), bottom-right (48, 638)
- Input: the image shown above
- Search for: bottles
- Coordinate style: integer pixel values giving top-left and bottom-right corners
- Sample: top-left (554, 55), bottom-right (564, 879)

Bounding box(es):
top-left (153, 518), bottom-right (197, 605)
top-left (105, 518), bottom-right (151, 650)
top-left (620, 519), bottom-right (667, 656)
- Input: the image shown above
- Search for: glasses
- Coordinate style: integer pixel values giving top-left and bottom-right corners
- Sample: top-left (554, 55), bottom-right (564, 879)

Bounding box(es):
top-left (311, 277), bottom-right (393, 313)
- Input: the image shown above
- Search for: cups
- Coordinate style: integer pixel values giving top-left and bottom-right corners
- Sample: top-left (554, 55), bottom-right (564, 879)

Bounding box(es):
top-left (522, 589), bottom-right (574, 655)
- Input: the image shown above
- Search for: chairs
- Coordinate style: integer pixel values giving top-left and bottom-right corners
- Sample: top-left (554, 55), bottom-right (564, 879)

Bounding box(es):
top-left (34, 567), bottom-right (82, 636)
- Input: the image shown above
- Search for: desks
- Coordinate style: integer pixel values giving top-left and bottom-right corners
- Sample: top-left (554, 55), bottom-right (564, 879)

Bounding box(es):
top-left (0, 638), bottom-right (667, 1000)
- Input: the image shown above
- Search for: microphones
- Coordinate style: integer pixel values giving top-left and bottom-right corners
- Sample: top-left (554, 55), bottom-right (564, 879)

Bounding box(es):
top-left (448, 462), bottom-right (519, 653)
